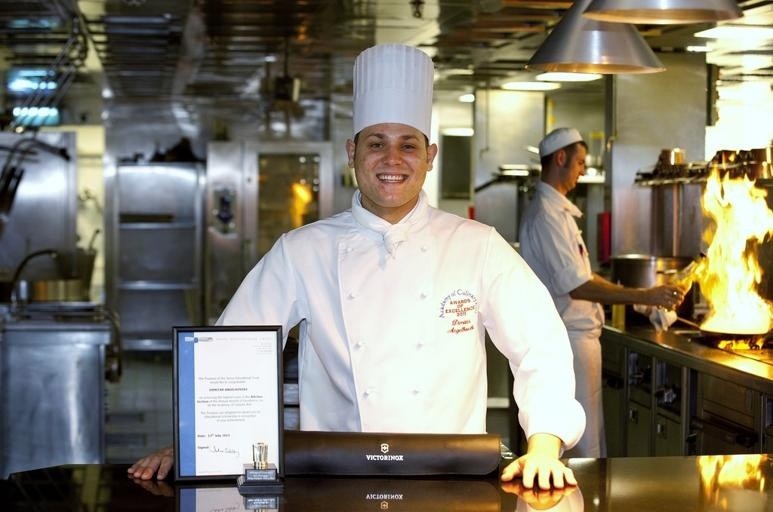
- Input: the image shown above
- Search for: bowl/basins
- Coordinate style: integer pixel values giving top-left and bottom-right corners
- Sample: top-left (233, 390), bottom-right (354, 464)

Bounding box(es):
top-left (652, 149), bottom-right (773, 181)
top-left (26, 277), bottom-right (90, 301)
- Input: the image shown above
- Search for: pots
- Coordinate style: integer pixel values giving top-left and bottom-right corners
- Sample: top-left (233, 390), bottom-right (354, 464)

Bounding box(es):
top-left (608, 252), bottom-right (696, 329)
top-left (675, 312), bottom-right (772, 346)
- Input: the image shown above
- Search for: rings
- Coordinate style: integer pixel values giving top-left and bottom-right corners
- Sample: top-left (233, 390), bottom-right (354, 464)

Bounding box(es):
top-left (672, 289), bottom-right (679, 298)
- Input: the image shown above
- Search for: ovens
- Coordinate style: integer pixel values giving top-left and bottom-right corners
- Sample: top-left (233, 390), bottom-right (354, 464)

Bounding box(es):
top-left (690, 409), bottom-right (762, 455)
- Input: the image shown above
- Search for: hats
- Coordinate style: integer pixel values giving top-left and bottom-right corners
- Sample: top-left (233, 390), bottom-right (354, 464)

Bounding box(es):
top-left (538, 127), bottom-right (584, 159)
top-left (352, 42), bottom-right (434, 145)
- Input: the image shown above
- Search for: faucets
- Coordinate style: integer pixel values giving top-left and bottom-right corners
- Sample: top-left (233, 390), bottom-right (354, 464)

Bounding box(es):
top-left (7, 249), bottom-right (72, 304)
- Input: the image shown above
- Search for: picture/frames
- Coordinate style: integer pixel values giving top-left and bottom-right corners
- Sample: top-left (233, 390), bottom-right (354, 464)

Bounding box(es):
top-left (169, 325), bottom-right (285, 484)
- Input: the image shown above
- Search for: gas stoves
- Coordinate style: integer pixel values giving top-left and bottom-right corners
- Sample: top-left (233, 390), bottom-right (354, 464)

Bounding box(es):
top-left (693, 335), bottom-right (773, 351)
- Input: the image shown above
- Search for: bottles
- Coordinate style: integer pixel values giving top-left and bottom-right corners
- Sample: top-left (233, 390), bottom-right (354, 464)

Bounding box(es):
top-left (610, 279), bottom-right (627, 330)
top-left (588, 129), bottom-right (606, 173)
top-left (656, 252), bottom-right (707, 311)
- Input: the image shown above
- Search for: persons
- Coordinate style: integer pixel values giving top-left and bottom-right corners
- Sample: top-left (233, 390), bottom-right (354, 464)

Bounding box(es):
top-left (133, 480), bottom-right (587, 512)
top-left (122, 122), bottom-right (587, 493)
top-left (515, 125), bottom-right (688, 456)
